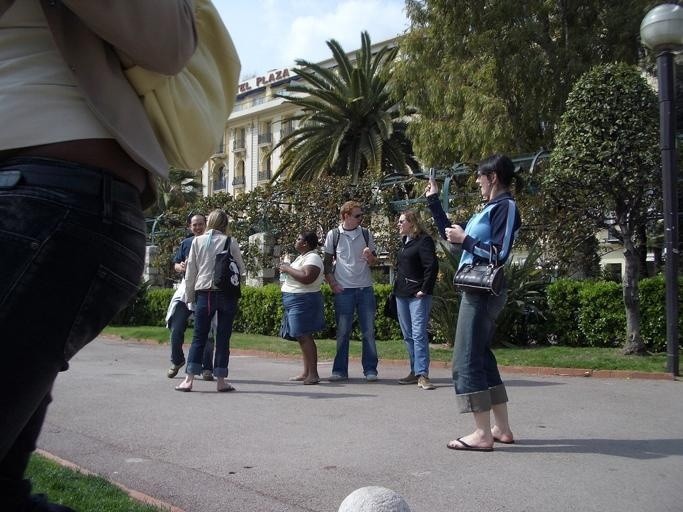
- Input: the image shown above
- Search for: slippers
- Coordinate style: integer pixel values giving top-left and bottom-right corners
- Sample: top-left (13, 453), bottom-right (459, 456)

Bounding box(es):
top-left (447, 437), bottom-right (493, 451)
top-left (175, 383), bottom-right (191, 392)
top-left (219, 383), bottom-right (235, 391)
top-left (493, 437), bottom-right (514, 444)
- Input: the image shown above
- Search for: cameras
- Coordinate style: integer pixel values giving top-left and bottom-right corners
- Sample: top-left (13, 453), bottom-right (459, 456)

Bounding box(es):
top-left (427, 167), bottom-right (439, 179)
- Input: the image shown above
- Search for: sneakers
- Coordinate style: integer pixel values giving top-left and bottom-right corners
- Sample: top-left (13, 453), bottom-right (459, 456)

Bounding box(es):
top-left (366, 374), bottom-right (377, 381)
top-left (328, 375), bottom-right (347, 381)
top-left (168, 361), bottom-right (185, 378)
top-left (418, 376), bottom-right (435, 389)
top-left (289, 374), bottom-right (306, 380)
top-left (202, 369), bottom-right (213, 380)
top-left (398, 373), bottom-right (418, 384)
top-left (303, 376), bottom-right (320, 385)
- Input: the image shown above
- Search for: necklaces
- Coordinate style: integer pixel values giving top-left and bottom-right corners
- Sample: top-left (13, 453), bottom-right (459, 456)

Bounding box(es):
top-left (300, 250), bottom-right (313, 257)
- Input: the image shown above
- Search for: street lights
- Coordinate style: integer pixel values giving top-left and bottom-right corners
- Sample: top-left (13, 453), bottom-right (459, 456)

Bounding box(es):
top-left (634, 2), bottom-right (683, 372)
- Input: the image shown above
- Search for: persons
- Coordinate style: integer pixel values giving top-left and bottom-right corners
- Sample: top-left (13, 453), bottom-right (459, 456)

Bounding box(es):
top-left (424, 152), bottom-right (524, 453)
top-left (322, 198), bottom-right (380, 385)
top-left (279, 228), bottom-right (326, 385)
top-left (175, 208), bottom-right (246, 395)
top-left (0, 0), bottom-right (245, 511)
top-left (389, 208), bottom-right (439, 392)
top-left (165, 212), bottom-right (219, 382)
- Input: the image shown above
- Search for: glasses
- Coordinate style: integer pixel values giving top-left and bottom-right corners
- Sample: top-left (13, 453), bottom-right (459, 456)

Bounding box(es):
top-left (352, 213), bottom-right (364, 218)
top-left (476, 173), bottom-right (484, 178)
top-left (398, 220), bottom-right (405, 223)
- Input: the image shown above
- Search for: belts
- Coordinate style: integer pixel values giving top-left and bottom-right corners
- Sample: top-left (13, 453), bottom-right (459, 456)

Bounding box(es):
top-left (11, 138), bottom-right (147, 194)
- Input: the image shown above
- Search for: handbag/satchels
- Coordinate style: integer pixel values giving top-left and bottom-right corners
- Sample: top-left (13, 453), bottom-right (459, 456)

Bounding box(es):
top-left (281, 313), bottom-right (297, 341)
top-left (453, 245), bottom-right (504, 296)
top-left (215, 251), bottom-right (240, 297)
top-left (384, 295), bottom-right (397, 316)
top-left (125, 0), bottom-right (240, 172)
